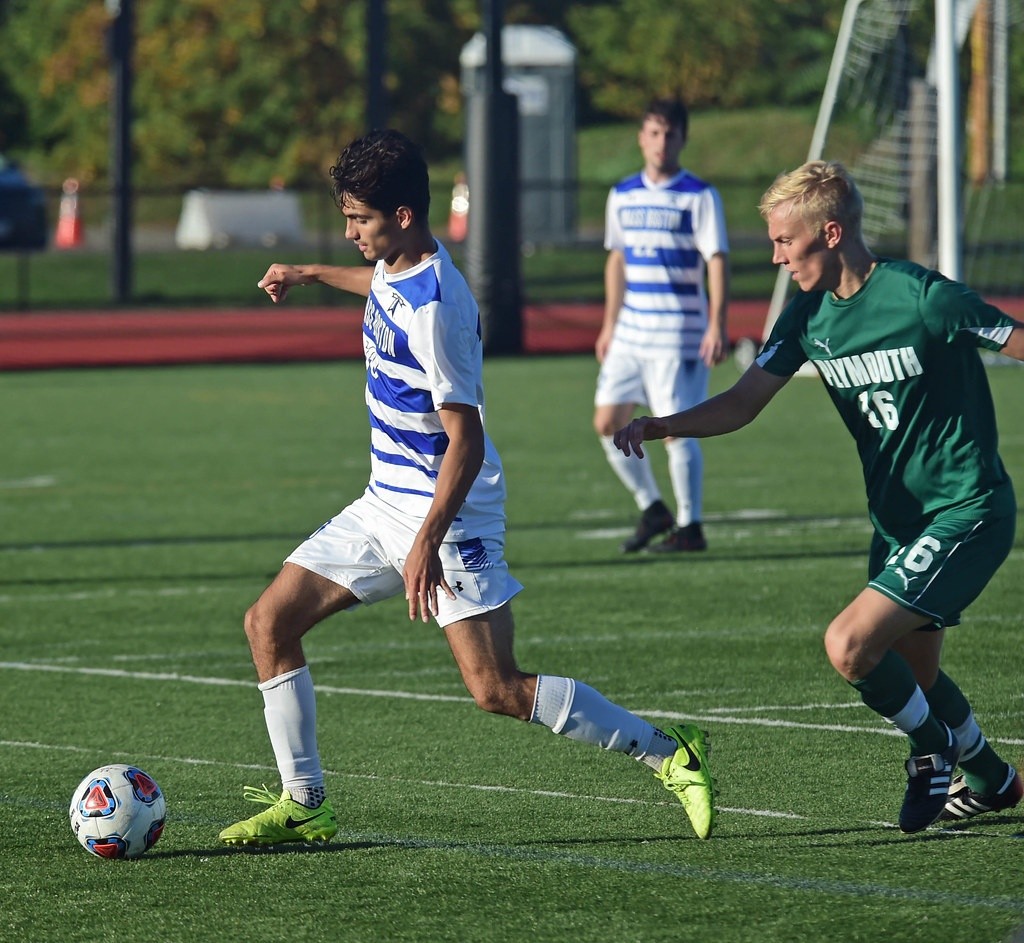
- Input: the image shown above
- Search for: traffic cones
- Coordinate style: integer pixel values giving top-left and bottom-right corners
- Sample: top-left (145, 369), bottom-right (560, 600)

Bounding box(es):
top-left (56, 176), bottom-right (85, 253)
top-left (447, 169), bottom-right (470, 245)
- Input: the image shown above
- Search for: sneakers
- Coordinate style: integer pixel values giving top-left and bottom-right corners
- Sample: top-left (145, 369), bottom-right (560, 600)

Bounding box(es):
top-left (929, 762), bottom-right (1023, 825)
top-left (653, 724), bottom-right (720, 840)
top-left (218, 783), bottom-right (337, 850)
top-left (646, 521), bottom-right (707, 554)
top-left (897, 718), bottom-right (961, 834)
top-left (620, 499), bottom-right (675, 552)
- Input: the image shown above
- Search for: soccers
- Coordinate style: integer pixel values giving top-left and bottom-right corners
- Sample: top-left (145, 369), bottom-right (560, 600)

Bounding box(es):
top-left (68, 763), bottom-right (166, 861)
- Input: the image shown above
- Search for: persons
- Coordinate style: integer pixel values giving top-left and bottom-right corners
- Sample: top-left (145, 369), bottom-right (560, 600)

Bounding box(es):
top-left (610, 159), bottom-right (1024, 831)
top-left (219, 129), bottom-right (728, 854)
top-left (595, 96), bottom-right (729, 556)
